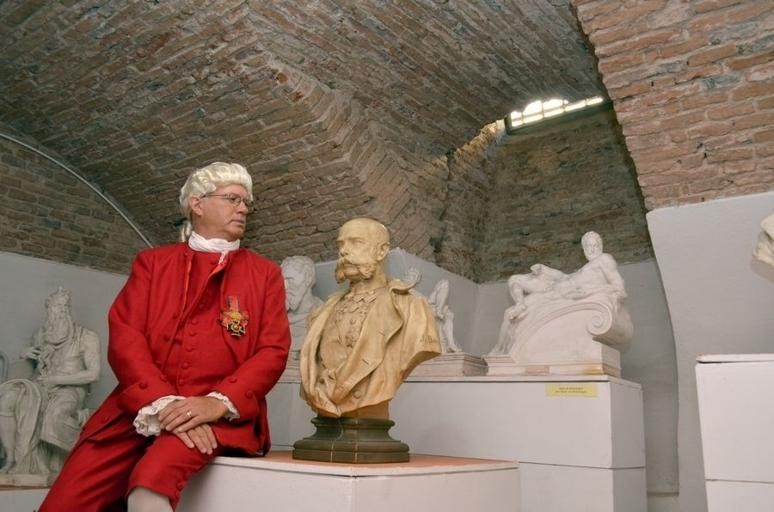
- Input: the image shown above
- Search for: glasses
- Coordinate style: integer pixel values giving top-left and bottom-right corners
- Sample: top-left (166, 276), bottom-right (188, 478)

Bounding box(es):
top-left (206, 192), bottom-right (254, 212)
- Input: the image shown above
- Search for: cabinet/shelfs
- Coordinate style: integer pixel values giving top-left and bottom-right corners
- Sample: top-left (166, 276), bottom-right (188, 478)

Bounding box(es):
top-left (269, 383), bottom-right (647, 512)
top-left (695, 355), bottom-right (772, 510)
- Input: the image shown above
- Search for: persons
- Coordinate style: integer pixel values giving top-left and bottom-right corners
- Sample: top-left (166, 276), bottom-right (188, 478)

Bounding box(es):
top-left (401, 266), bottom-right (463, 353)
top-left (298, 216), bottom-right (441, 418)
top-left (278, 254), bottom-right (325, 360)
top-left (1, 283), bottom-right (102, 474)
top-left (38, 161), bottom-right (291, 511)
top-left (476, 229), bottom-right (628, 359)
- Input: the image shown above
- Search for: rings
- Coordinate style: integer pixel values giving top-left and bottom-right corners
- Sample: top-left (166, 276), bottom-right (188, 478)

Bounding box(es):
top-left (186, 409), bottom-right (192, 417)
